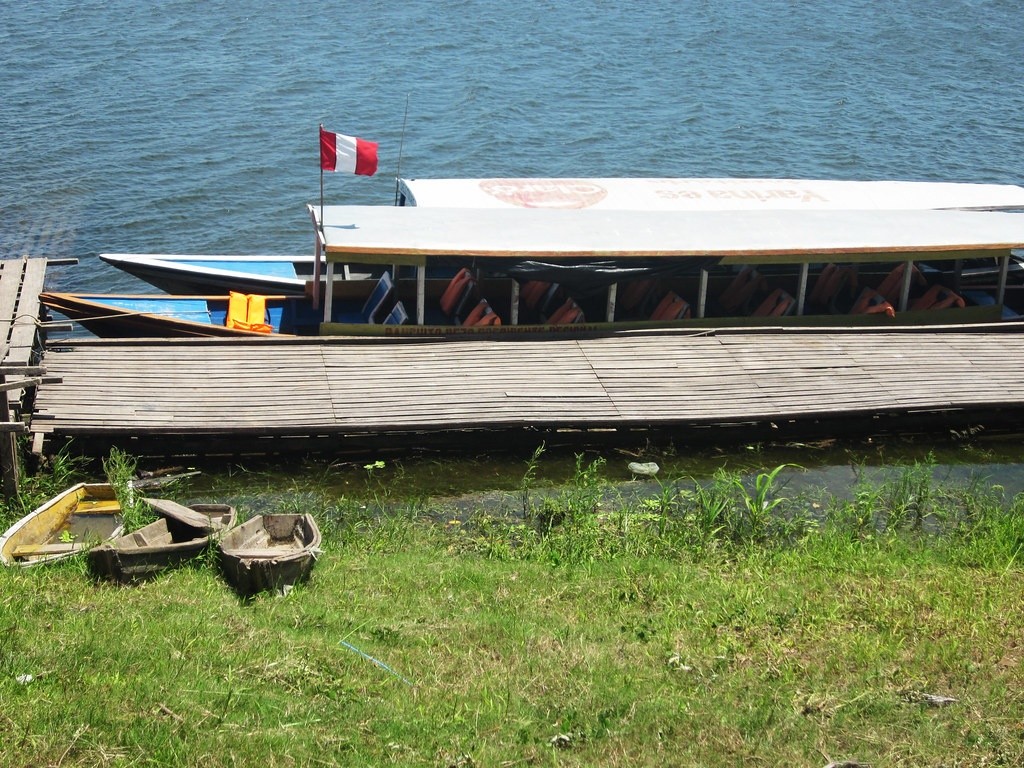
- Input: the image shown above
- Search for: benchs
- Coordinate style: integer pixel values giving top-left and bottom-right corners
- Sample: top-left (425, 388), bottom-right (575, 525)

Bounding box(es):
top-left (73, 501), bottom-right (120, 515)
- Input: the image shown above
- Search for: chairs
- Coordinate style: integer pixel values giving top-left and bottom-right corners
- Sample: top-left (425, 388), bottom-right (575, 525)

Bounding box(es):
top-left (335, 264), bottom-right (965, 325)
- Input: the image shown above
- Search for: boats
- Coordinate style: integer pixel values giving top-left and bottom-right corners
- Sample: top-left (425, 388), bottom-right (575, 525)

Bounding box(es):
top-left (217, 508), bottom-right (323, 600)
top-left (88, 504), bottom-right (240, 584)
top-left (0, 481), bottom-right (138, 572)
top-left (35, 176), bottom-right (1024, 343)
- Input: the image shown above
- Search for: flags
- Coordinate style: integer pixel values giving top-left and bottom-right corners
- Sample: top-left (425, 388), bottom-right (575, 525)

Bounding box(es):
top-left (321, 130), bottom-right (377, 176)
